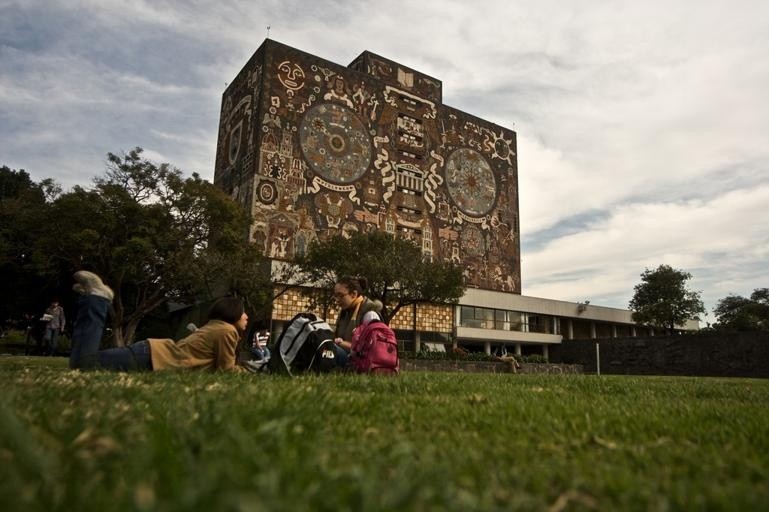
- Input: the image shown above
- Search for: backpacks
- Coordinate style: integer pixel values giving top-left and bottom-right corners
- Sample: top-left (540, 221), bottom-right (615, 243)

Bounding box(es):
top-left (264, 310), bottom-right (338, 379)
top-left (348, 318), bottom-right (401, 377)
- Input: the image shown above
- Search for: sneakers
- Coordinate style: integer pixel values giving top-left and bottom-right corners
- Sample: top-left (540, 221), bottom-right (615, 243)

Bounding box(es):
top-left (71, 269), bottom-right (115, 301)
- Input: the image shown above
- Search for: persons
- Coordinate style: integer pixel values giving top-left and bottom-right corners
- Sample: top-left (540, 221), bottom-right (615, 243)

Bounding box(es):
top-left (306, 274), bottom-right (388, 373)
top-left (493, 341), bottom-right (521, 373)
top-left (65, 269), bottom-right (249, 376)
top-left (250, 324), bottom-right (273, 360)
top-left (44, 298), bottom-right (66, 355)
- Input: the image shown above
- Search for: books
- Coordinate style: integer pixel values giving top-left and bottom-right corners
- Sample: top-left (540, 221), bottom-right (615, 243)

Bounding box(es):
top-left (40, 313), bottom-right (54, 322)
